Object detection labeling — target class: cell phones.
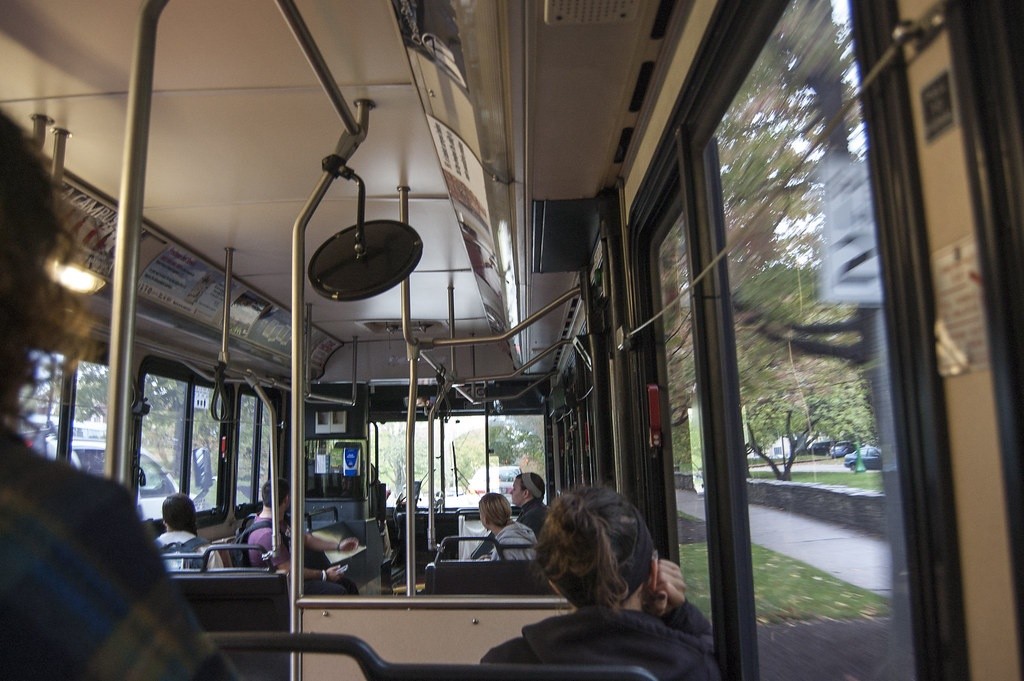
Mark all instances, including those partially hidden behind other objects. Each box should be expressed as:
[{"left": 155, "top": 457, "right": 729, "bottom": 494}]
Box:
[{"left": 336, "top": 564, "right": 348, "bottom": 572}]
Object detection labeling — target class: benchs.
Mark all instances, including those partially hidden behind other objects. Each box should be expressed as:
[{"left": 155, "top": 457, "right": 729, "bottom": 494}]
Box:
[{"left": 156, "top": 507, "right": 662, "bottom": 681}]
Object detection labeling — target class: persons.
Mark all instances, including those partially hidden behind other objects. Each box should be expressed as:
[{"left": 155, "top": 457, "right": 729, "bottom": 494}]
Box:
[
  {"left": 155, "top": 493, "right": 224, "bottom": 570},
  {"left": 479, "top": 484, "right": 719, "bottom": 681},
  {"left": 249, "top": 477, "right": 360, "bottom": 596},
  {"left": 415, "top": 396, "right": 430, "bottom": 407},
  {"left": 0, "top": 100, "right": 239, "bottom": 681},
  {"left": 476, "top": 472, "right": 549, "bottom": 560}
]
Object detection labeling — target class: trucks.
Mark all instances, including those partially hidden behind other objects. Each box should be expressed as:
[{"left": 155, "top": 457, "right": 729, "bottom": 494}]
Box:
[{"left": 25, "top": 411, "right": 214, "bottom": 520}]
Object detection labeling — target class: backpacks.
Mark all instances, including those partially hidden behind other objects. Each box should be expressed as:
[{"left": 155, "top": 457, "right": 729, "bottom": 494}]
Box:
[
  {"left": 232, "top": 521, "right": 289, "bottom": 569},
  {"left": 154, "top": 537, "right": 207, "bottom": 569}
]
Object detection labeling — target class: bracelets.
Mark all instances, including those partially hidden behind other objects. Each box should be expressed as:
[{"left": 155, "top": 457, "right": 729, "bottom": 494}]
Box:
[
  {"left": 322, "top": 569, "right": 327, "bottom": 582},
  {"left": 337, "top": 543, "right": 340, "bottom": 552}
]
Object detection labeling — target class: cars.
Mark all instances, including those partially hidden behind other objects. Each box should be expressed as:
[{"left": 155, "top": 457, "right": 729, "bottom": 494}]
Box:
[
  {"left": 807, "top": 440, "right": 834, "bottom": 456},
  {"left": 829, "top": 440, "right": 856, "bottom": 458},
  {"left": 447, "top": 486, "right": 464, "bottom": 497},
  {"left": 467, "top": 465, "right": 524, "bottom": 507},
  {"left": 843, "top": 446, "right": 883, "bottom": 471}
]
